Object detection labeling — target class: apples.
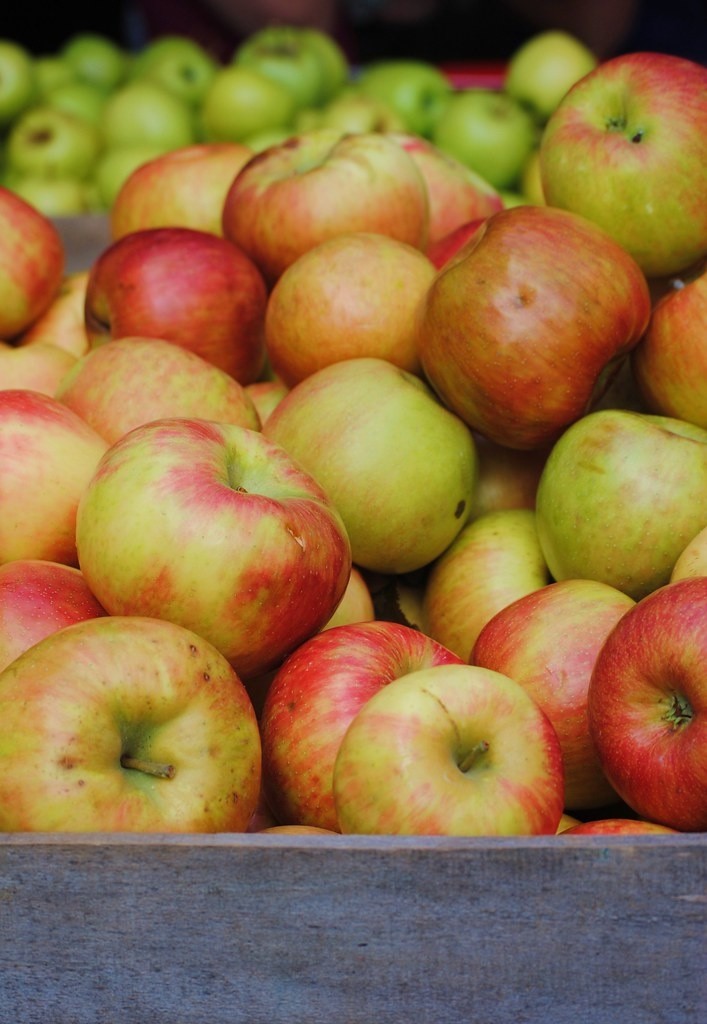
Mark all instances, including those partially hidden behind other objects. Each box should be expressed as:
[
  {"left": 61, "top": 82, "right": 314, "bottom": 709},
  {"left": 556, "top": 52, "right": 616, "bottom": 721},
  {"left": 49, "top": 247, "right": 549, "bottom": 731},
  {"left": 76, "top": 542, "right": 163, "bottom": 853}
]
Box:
[{"left": 0, "top": 23, "right": 707, "bottom": 838}]
